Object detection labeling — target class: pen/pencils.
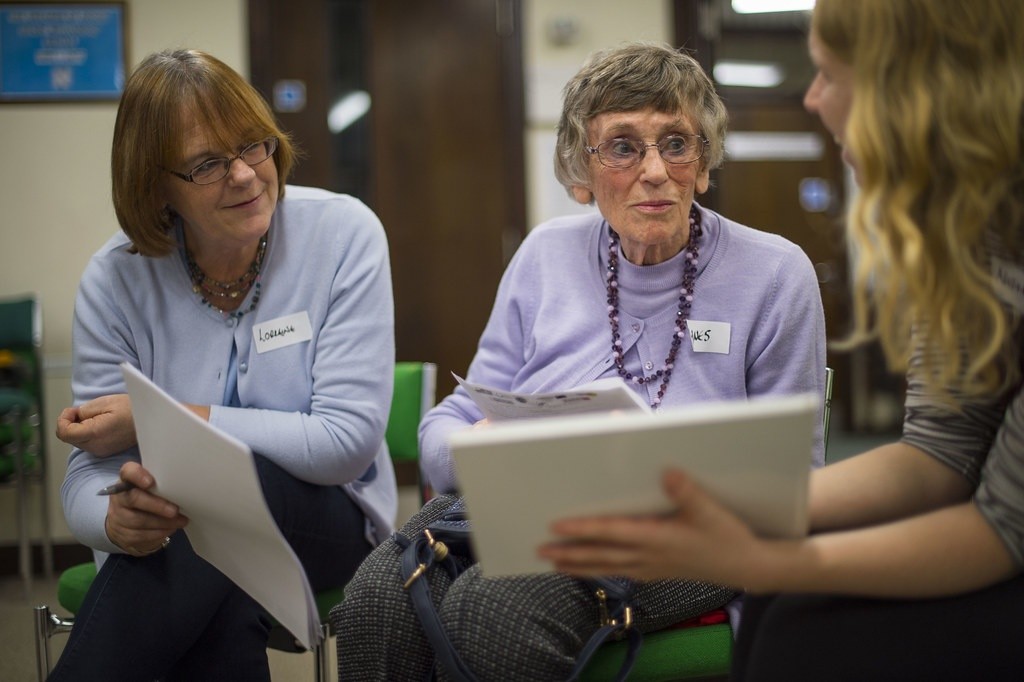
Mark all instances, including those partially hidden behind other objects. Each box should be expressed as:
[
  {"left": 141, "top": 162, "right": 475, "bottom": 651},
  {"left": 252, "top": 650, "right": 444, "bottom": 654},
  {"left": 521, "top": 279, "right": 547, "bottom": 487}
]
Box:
[{"left": 96, "top": 480, "right": 132, "bottom": 495}]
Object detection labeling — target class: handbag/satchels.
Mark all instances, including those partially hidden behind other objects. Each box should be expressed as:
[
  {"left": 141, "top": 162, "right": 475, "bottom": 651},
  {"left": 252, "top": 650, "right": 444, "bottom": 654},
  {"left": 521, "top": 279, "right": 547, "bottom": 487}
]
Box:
[{"left": 400, "top": 499, "right": 471, "bottom": 581}]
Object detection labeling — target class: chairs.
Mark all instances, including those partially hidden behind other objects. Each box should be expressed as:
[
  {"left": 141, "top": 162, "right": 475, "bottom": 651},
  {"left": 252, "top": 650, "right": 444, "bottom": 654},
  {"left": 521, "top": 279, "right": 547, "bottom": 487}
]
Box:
[
  {"left": 0, "top": 291, "right": 52, "bottom": 600},
  {"left": 33, "top": 358, "right": 836, "bottom": 681}
]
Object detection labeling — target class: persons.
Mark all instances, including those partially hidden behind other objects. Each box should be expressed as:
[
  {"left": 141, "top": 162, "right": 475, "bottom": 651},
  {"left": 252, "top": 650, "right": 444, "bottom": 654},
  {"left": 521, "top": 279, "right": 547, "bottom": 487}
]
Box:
[
  {"left": 44, "top": 49, "right": 397, "bottom": 682},
  {"left": 540, "top": 0, "right": 1024, "bottom": 682},
  {"left": 338, "top": 43, "right": 829, "bottom": 682}
]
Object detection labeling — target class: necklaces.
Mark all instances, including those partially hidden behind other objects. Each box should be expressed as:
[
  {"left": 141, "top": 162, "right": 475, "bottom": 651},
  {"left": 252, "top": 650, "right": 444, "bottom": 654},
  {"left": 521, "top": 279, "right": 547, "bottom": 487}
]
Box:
[
  {"left": 607, "top": 207, "right": 700, "bottom": 414},
  {"left": 177, "top": 221, "right": 267, "bottom": 318}
]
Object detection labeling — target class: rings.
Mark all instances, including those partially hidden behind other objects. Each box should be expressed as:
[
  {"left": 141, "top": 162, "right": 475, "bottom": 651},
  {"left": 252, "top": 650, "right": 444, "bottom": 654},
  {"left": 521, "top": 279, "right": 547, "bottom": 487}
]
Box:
[{"left": 162, "top": 537, "right": 170, "bottom": 546}]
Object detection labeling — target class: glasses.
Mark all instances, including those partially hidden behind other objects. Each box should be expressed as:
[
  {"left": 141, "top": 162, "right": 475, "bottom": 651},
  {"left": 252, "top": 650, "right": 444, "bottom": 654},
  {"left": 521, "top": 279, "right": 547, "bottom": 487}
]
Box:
[
  {"left": 585, "top": 132, "right": 710, "bottom": 169},
  {"left": 162, "top": 134, "right": 278, "bottom": 186}
]
